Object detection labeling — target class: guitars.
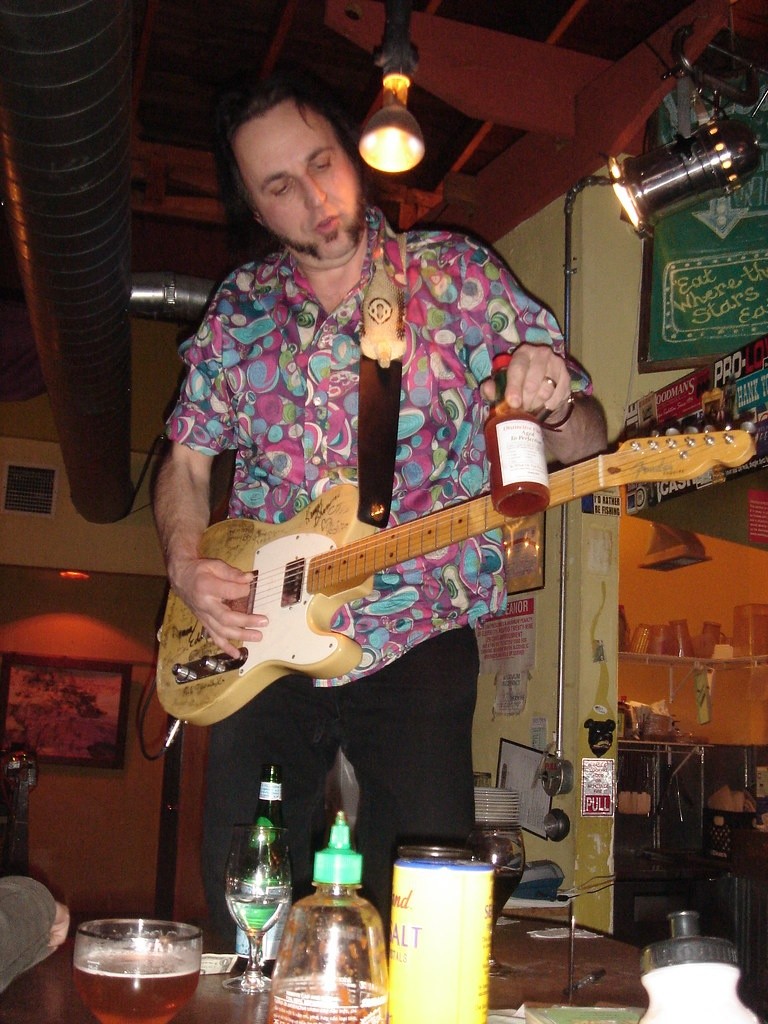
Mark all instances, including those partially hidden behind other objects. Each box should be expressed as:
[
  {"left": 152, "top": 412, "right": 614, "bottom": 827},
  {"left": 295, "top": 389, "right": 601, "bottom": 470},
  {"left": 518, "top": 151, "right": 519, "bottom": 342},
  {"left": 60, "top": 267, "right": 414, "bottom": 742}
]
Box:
[{"left": 153, "top": 420, "right": 756, "bottom": 726}]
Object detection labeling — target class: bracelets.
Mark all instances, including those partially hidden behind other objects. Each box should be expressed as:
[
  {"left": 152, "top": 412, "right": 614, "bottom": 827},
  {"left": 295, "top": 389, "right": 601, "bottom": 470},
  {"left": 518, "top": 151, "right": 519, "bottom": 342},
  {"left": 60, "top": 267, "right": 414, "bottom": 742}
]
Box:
[{"left": 542, "top": 391, "right": 575, "bottom": 429}]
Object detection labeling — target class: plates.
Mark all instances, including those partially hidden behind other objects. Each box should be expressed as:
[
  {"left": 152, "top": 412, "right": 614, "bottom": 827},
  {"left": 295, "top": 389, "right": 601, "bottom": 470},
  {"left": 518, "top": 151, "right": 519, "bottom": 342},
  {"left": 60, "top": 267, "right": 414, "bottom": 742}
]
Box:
[{"left": 474, "top": 785, "right": 521, "bottom": 821}]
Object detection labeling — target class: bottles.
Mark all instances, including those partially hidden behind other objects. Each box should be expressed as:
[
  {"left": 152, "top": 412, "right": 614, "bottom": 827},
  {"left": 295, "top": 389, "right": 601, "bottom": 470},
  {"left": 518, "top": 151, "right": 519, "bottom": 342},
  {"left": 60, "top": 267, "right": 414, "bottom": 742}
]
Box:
[
  {"left": 619, "top": 605, "right": 627, "bottom": 652},
  {"left": 698, "top": 621, "right": 723, "bottom": 658},
  {"left": 265, "top": 810, "right": 389, "bottom": 1024},
  {"left": 636, "top": 910, "right": 760, "bottom": 1024},
  {"left": 484, "top": 353, "right": 551, "bottom": 516},
  {"left": 235, "top": 764, "right": 297, "bottom": 978}
]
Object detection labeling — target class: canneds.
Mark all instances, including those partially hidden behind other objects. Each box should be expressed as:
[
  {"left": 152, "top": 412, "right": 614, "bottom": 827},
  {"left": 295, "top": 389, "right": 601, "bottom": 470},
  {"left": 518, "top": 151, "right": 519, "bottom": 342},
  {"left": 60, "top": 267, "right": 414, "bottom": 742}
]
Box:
[{"left": 386, "top": 858, "right": 494, "bottom": 1024}]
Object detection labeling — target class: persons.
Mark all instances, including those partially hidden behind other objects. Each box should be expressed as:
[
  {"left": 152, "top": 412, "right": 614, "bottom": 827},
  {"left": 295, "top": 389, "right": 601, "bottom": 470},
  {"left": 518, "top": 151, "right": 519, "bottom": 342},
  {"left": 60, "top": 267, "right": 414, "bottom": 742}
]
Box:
[{"left": 153, "top": 71, "right": 608, "bottom": 980}]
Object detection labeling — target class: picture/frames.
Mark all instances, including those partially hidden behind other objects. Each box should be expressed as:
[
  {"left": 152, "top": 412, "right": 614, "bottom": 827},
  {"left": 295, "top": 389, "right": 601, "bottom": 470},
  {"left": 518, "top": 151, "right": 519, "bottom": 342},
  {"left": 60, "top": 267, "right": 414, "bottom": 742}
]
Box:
[{"left": 0, "top": 650, "right": 133, "bottom": 770}]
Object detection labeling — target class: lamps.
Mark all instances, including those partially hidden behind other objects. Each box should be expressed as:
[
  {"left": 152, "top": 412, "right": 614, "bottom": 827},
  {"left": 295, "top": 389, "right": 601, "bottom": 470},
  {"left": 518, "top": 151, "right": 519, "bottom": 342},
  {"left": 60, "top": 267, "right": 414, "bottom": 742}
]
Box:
[
  {"left": 603, "top": 117, "right": 762, "bottom": 238},
  {"left": 354, "top": 0, "right": 424, "bottom": 173}
]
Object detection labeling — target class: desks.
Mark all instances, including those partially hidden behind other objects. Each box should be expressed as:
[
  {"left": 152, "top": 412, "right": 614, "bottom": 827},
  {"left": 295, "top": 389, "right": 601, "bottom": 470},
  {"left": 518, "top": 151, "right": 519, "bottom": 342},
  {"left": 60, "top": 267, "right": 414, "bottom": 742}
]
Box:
[{"left": 0, "top": 913, "right": 650, "bottom": 1024}]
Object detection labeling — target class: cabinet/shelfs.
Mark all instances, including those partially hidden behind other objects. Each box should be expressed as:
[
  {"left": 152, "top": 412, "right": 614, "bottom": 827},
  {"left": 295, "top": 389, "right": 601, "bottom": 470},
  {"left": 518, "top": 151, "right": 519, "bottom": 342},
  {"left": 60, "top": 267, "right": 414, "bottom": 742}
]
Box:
[{"left": 614, "top": 739, "right": 768, "bottom": 1023}]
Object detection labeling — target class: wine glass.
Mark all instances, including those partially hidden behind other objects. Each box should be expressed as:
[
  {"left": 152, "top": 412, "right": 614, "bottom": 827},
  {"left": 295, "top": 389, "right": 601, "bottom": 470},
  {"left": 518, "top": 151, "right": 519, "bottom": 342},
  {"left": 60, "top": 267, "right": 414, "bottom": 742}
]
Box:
[
  {"left": 467, "top": 826, "right": 525, "bottom": 976},
  {"left": 223, "top": 823, "right": 292, "bottom": 996}
]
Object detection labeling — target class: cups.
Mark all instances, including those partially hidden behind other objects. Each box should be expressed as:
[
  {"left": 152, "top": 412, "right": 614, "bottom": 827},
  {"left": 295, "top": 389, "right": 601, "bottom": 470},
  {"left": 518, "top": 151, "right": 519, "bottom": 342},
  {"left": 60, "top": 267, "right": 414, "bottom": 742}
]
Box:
[
  {"left": 733, "top": 604, "right": 768, "bottom": 657},
  {"left": 663, "top": 618, "right": 697, "bottom": 657},
  {"left": 384, "top": 844, "right": 496, "bottom": 1024},
  {"left": 72, "top": 917, "right": 204, "bottom": 1024},
  {"left": 629, "top": 624, "right": 652, "bottom": 655},
  {"left": 648, "top": 624, "right": 668, "bottom": 655}
]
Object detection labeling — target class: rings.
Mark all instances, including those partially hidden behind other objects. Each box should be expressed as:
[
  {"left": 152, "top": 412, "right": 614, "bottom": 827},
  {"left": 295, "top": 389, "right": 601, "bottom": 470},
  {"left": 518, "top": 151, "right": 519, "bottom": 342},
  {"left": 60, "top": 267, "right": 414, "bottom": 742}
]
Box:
[{"left": 543, "top": 376, "right": 557, "bottom": 389}]
